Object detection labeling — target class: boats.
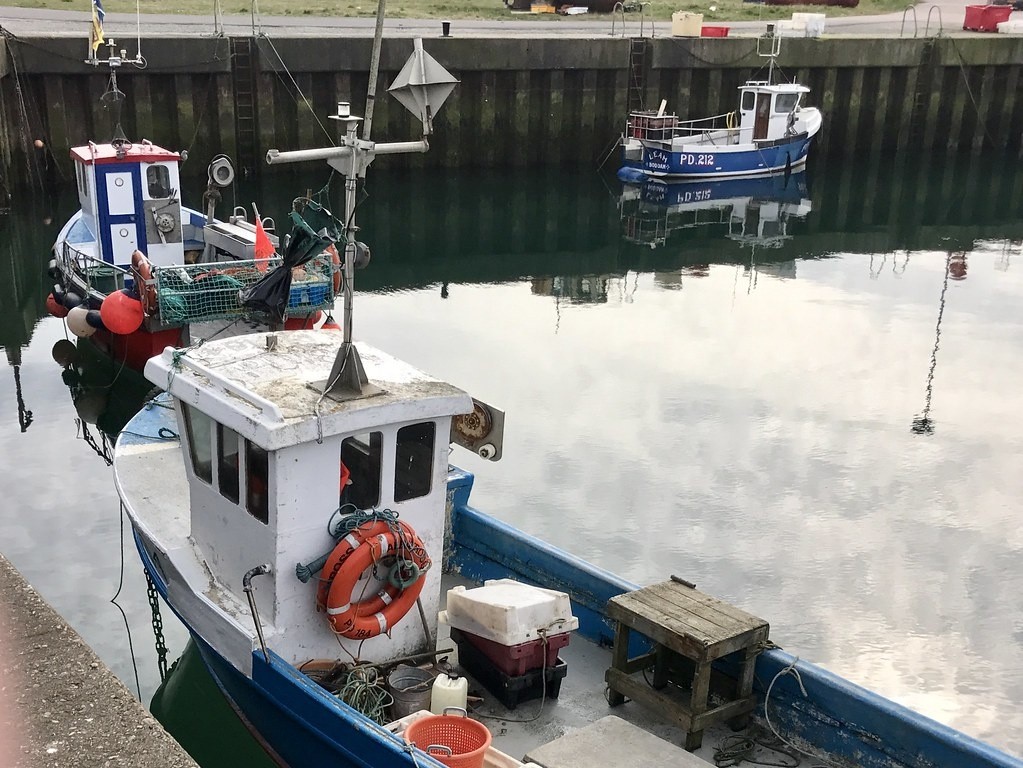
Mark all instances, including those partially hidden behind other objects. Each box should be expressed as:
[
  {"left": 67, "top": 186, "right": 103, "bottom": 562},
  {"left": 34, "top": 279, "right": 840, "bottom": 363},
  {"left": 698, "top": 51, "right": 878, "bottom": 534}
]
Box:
[
  {"left": 615, "top": 20, "right": 820, "bottom": 189},
  {"left": 45, "top": 108, "right": 347, "bottom": 385},
  {"left": 617, "top": 193, "right": 812, "bottom": 268},
  {"left": 113, "top": 98, "right": 1023, "bottom": 767}
]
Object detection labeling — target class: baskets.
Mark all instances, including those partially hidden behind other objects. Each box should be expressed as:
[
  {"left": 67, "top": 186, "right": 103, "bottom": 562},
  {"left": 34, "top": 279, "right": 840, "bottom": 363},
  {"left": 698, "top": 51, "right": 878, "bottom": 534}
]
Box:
[{"left": 404, "top": 707, "right": 492, "bottom": 768}]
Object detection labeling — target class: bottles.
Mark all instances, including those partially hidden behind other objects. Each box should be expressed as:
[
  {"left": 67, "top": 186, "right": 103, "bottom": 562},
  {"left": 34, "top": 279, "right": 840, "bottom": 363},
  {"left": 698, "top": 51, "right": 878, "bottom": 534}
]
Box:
[{"left": 430, "top": 672, "right": 469, "bottom": 716}]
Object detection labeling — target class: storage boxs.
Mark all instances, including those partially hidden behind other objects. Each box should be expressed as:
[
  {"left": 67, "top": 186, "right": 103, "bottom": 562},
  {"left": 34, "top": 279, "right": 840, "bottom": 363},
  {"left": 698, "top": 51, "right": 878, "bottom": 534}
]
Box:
[
  {"left": 701, "top": 26, "right": 731, "bottom": 38},
  {"left": 384, "top": 708, "right": 527, "bottom": 768},
  {"left": 996, "top": 21, "right": 1023, "bottom": 34},
  {"left": 672, "top": 10, "right": 704, "bottom": 36},
  {"left": 776, "top": 12, "right": 827, "bottom": 39},
  {"left": 439, "top": 578, "right": 580, "bottom": 709},
  {"left": 962, "top": 4, "right": 1014, "bottom": 33},
  {"left": 291, "top": 286, "right": 328, "bottom": 308}
]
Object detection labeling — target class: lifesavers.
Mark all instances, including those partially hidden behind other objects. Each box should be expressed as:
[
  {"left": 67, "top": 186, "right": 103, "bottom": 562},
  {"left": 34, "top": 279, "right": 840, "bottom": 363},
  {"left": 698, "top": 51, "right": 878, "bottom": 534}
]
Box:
[
  {"left": 325, "top": 243, "right": 341, "bottom": 298},
  {"left": 317, "top": 519, "right": 416, "bottom": 617},
  {"left": 132, "top": 249, "right": 156, "bottom": 316},
  {"left": 327, "top": 531, "right": 431, "bottom": 639}
]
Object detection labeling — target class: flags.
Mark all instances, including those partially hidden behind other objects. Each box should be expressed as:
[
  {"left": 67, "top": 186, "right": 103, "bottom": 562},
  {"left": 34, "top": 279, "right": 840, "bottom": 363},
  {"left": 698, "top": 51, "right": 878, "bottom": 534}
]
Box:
[{"left": 254, "top": 214, "right": 275, "bottom": 271}]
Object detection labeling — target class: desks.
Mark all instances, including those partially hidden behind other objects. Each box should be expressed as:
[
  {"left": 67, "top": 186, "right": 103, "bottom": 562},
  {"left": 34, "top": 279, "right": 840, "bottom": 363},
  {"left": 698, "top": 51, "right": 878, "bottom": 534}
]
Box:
[{"left": 605, "top": 578, "right": 769, "bottom": 753}]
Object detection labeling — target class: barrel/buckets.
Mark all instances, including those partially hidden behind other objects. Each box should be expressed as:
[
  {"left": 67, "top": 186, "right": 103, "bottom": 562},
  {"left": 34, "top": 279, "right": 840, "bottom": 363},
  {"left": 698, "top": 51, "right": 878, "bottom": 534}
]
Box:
[{"left": 386, "top": 664, "right": 436, "bottom": 721}]
